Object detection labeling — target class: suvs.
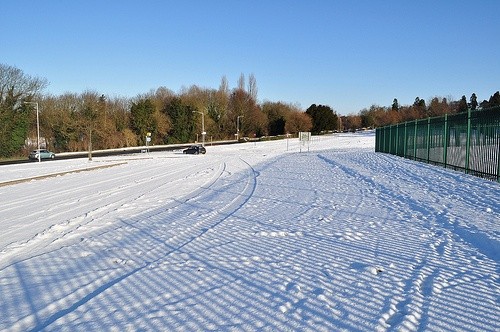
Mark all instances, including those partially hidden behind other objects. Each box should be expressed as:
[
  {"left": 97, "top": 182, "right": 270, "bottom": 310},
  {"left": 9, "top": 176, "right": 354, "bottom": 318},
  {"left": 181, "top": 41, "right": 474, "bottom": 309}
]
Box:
[{"left": 183, "top": 145, "right": 206, "bottom": 155}]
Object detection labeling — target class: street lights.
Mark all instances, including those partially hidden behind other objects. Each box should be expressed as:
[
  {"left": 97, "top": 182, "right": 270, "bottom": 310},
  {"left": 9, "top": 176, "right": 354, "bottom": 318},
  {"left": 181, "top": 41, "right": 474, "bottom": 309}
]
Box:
[
  {"left": 237, "top": 116, "right": 244, "bottom": 141},
  {"left": 23, "top": 101, "right": 41, "bottom": 163},
  {"left": 192, "top": 111, "right": 205, "bottom": 147}
]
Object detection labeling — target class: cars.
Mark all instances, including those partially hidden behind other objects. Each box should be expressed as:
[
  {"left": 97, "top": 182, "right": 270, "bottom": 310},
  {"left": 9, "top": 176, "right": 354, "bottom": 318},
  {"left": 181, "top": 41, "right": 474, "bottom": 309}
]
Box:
[{"left": 29, "top": 149, "right": 55, "bottom": 160}]
